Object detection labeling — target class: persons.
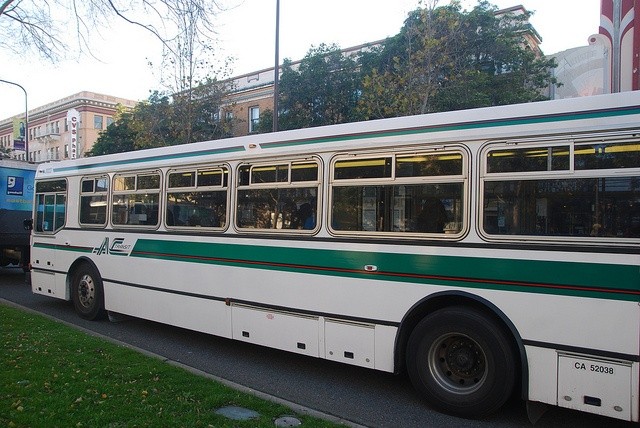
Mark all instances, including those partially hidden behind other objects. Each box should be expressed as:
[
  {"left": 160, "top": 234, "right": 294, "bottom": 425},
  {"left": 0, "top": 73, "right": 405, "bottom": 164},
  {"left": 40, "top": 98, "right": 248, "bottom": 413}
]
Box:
[{"left": 304, "top": 196, "right": 339, "bottom": 229}]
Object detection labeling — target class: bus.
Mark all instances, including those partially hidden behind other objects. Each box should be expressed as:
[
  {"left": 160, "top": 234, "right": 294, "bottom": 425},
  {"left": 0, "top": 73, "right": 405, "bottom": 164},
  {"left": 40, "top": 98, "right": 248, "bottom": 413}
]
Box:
[{"left": 28, "top": 90, "right": 640, "bottom": 423}]
